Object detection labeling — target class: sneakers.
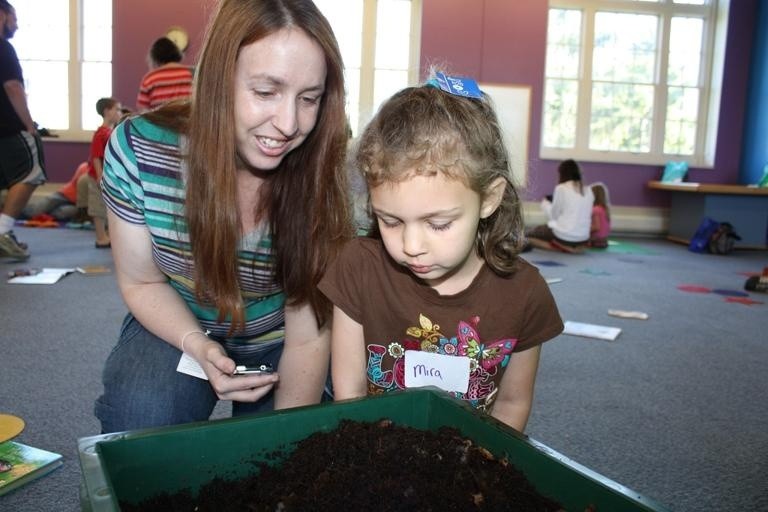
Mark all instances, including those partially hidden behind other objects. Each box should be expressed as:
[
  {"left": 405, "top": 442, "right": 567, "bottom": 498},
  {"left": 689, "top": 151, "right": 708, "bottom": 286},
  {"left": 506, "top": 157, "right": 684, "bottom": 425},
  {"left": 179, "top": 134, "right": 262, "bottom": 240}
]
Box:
[
  {"left": 96, "top": 239, "right": 111, "bottom": 249},
  {"left": 0, "top": 230, "right": 31, "bottom": 261}
]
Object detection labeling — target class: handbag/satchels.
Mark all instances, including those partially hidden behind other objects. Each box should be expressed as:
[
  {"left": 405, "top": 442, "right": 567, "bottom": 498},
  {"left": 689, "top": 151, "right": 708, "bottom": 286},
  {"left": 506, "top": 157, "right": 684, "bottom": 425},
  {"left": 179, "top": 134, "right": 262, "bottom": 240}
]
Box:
[{"left": 688, "top": 216, "right": 740, "bottom": 255}]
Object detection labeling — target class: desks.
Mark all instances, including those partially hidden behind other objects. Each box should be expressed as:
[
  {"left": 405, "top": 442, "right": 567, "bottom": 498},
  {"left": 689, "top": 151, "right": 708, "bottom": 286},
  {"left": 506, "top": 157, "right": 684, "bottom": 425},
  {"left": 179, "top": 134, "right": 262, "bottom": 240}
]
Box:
[{"left": 646, "top": 180, "right": 767, "bottom": 250}]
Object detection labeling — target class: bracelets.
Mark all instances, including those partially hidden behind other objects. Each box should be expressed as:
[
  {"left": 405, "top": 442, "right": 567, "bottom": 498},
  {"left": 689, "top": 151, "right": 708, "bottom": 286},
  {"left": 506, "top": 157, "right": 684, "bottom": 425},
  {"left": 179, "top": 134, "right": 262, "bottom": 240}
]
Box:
[{"left": 179, "top": 326, "right": 209, "bottom": 356}]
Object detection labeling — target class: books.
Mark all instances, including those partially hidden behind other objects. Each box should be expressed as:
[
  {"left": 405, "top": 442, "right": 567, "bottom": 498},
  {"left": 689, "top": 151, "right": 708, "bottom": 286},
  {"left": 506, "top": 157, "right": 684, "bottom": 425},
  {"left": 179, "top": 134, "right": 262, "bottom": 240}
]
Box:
[
  {"left": 6, "top": 267, "right": 76, "bottom": 285},
  {"left": 0, "top": 438, "right": 64, "bottom": 498}
]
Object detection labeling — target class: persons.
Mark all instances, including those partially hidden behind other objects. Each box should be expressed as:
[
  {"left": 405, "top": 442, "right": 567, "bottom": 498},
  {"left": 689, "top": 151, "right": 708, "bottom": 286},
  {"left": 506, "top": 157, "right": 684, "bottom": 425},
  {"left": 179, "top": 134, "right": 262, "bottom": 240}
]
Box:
[
  {"left": 133, "top": 35, "right": 198, "bottom": 112},
  {"left": 84, "top": 97, "right": 126, "bottom": 248},
  {"left": 1, "top": 157, "right": 96, "bottom": 227},
  {"left": 587, "top": 181, "right": 611, "bottom": 251},
  {"left": 91, "top": 0, "right": 354, "bottom": 431},
  {"left": 521, "top": 158, "right": 594, "bottom": 254},
  {"left": 1, "top": 0, "right": 45, "bottom": 265},
  {"left": 309, "top": 64, "right": 565, "bottom": 442}
]
